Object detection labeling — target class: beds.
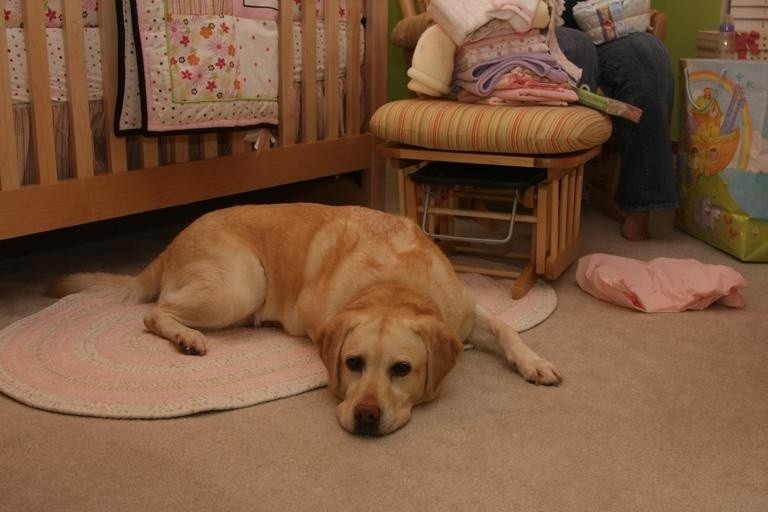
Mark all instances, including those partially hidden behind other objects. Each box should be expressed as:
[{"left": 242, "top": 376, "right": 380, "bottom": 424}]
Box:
[{"left": 0, "top": 2, "right": 393, "bottom": 258}]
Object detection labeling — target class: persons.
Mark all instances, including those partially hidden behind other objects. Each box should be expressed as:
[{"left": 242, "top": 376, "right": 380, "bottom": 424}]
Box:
[{"left": 546, "top": 0, "right": 683, "bottom": 242}]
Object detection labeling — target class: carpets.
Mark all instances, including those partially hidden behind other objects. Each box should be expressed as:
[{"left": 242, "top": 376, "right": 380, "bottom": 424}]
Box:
[{"left": 2, "top": 263, "right": 559, "bottom": 419}]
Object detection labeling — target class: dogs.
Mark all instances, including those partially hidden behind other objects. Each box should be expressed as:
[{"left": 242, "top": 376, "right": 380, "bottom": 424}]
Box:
[{"left": 41, "top": 197, "right": 565, "bottom": 440}]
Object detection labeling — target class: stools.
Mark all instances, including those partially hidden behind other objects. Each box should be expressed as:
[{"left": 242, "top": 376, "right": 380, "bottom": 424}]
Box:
[{"left": 372, "top": 94, "right": 617, "bottom": 287}]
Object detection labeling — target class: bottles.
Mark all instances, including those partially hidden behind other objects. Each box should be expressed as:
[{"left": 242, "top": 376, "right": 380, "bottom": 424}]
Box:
[{"left": 719, "top": 23, "right": 736, "bottom": 60}]
